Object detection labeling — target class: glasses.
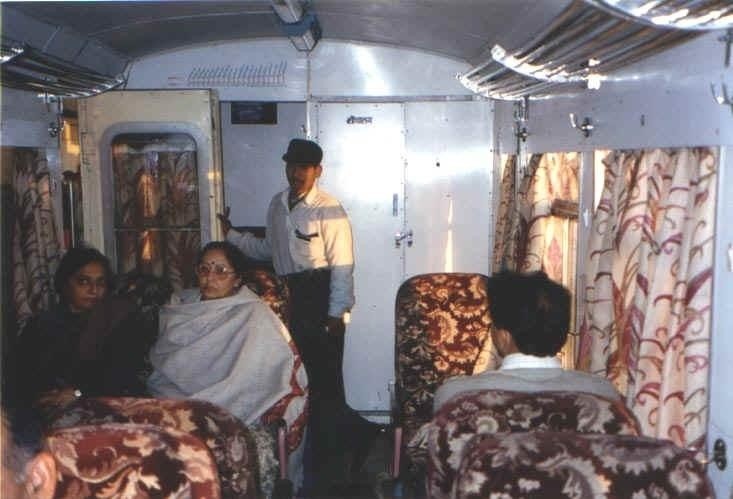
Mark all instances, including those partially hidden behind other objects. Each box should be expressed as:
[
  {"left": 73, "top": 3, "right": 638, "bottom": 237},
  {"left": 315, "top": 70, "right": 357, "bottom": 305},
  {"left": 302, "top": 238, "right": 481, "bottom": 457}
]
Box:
[{"left": 196, "top": 264, "right": 238, "bottom": 276}]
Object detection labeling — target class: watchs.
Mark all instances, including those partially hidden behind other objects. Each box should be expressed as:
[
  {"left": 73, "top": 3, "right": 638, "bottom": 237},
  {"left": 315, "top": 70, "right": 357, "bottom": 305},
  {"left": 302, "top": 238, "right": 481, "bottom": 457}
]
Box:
[{"left": 73, "top": 386, "right": 81, "bottom": 397}]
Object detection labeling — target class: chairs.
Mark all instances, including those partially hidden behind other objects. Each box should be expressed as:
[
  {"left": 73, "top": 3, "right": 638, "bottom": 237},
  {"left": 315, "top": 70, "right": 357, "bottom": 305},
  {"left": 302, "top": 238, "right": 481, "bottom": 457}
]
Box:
[
  {"left": 396, "top": 274, "right": 715, "bottom": 498},
  {"left": 2, "top": 267, "right": 308, "bottom": 495}
]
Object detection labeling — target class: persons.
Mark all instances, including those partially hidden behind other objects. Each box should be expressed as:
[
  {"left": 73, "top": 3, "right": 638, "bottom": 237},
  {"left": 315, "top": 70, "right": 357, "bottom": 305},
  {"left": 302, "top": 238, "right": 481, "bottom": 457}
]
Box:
[
  {"left": 148, "top": 241, "right": 296, "bottom": 497},
  {"left": 6, "top": 248, "right": 150, "bottom": 440},
  {"left": 424, "top": 271, "right": 627, "bottom": 428},
  {"left": 216, "top": 140, "right": 357, "bottom": 453}
]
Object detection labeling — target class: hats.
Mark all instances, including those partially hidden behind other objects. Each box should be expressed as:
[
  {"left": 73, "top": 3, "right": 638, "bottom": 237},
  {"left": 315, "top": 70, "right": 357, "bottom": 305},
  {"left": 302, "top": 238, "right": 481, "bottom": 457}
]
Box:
[{"left": 282, "top": 139, "right": 322, "bottom": 164}]
